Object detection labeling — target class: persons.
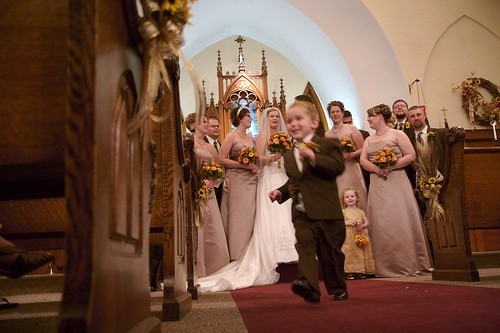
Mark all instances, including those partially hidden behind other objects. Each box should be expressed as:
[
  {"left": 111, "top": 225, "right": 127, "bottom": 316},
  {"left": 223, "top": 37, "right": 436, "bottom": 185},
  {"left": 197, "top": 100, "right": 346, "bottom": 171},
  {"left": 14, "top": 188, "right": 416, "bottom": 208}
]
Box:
[
  {"left": 185, "top": 107, "right": 300, "bottom": 292},
  {"left": 0, "top": 236, "right": 54, "bottom": 311},
  {"left": 341, "top": 187, "right": 376, "bottom": 280},
  {"left": 392, "top": 99, "right": 435, "bottom": 218},
  {"left": 269, "top": 95, "right": 370, "bottom": 303},
  {"left": 359, "top": 104, "right": 435, "bottom": 278}
]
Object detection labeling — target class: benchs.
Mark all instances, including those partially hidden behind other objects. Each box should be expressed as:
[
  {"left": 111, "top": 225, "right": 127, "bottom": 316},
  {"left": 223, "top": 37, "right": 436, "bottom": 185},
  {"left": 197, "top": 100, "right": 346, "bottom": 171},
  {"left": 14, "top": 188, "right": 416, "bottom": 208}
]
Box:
[
  {"left": 430, "top": 127, "right": 500, "bottom": 282},
  {"left": 0, "top": 0, "right": 200, "bottom": 333}
]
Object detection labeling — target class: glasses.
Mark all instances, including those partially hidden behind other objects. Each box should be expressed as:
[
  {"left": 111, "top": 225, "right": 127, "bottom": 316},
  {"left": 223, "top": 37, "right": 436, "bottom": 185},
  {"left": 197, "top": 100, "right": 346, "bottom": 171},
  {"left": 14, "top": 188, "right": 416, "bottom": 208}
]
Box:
[
  {"left": 245, "top": 113, "right": 251, "bottom": 117},
  {"left": 394, "top": 104, "right": 407, "bottom": 108}
]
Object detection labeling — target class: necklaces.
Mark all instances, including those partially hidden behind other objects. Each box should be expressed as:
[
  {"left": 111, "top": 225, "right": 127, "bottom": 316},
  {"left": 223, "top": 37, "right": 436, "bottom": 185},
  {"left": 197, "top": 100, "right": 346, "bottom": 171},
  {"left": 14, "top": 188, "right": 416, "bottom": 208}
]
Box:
[
  {"left": 195, "top": 138, "right": 206, "bottom": 146},
  {"left": 376, "top": 128, "right": 389, "bottom": 136},
  {"left": 332, "top": 125, "right": 343, "bottom": 134},
  {"left": 235, "top": 130, "right": 247, "bottom": 139}
]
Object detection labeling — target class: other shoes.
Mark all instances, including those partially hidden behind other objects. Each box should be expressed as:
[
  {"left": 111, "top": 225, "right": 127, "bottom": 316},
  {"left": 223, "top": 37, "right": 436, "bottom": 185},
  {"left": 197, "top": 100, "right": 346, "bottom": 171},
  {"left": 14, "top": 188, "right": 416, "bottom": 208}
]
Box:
[
  {"left": 291, "top": 281, "right": 320, "bottom": 304},
  {"left": 333, "top": 289, "right": 348, "bottom": 300},
  {"left": 347, "top": 272, "right": 367, "bottom": 279}
]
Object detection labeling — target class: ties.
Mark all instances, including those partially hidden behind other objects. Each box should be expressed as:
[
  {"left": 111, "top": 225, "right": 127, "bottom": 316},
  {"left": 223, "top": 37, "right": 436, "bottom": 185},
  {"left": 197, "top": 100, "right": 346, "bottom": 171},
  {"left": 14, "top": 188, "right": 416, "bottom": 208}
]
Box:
[
  {"left": 214, "top": 141, "right": 219, "bottom": 153},
  {"left": 295, "top": 141, "right": 305, "bottom": 166},
  {"left": 398, "top": 123, "right": 403, "bottom": 131},
  {"left": 417, "top": 132, "right": 424, "bottom": 148}
]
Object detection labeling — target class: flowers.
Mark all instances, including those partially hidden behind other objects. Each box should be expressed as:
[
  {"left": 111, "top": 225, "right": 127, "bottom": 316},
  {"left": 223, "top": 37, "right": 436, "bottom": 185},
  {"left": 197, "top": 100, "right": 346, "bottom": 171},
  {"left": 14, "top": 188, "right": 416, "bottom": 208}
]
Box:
[
  {"left": 415, "top": 175, "right": 446, "bottom": 223},
  {"left": 341, "top": 137, "right": 356, "bottom": 156},
  {"left": 266, "top": 131, "right": 293, "bottom": 168},
  {"left": 405, "top": 122, "right": 410, "bottom": 128},
  {"left": 238, "top": 146, "right": 260, "bottom": 176},
  {"left": 355, "top": 222, "right": 369, "bottom": 247},
  {"left": 373, "top": 144, "right": 399, "bottom": 180},
  {"left": 195, "top": 160, "right": 226, "bottom": 232}
]
